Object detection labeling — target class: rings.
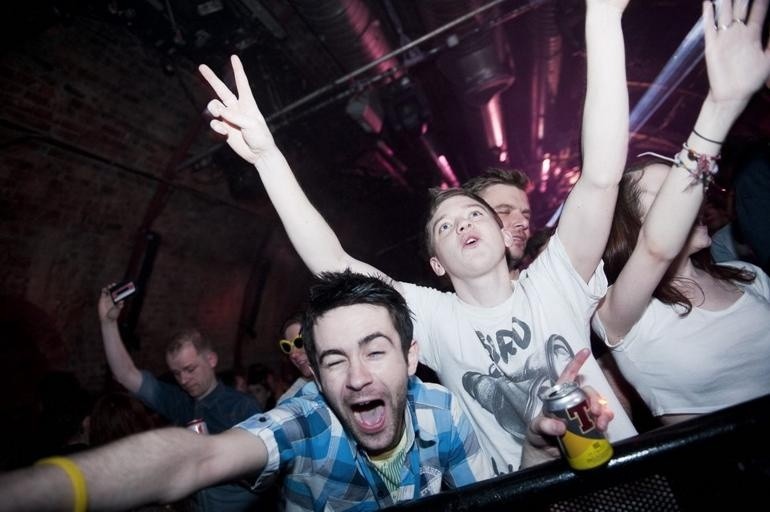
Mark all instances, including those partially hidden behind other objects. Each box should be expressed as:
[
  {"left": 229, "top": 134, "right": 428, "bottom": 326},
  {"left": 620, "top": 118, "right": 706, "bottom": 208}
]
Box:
[{"left": 714, "top": 17, "right": 749, "bottom": 30}]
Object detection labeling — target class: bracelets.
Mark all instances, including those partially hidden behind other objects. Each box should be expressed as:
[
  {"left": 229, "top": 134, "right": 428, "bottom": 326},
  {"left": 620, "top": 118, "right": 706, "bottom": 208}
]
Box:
[
  {"left": 674, "top": 127, "right": 726, "bottom": 188},
  {"left": 35, "top": 455, "right": 87, "bottom": 512}
]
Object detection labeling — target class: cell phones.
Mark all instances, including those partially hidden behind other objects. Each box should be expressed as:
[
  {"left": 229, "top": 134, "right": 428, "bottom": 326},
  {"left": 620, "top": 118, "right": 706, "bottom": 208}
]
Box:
[{"left": 109, "top": 276, "right": 137, "bottom": 304}]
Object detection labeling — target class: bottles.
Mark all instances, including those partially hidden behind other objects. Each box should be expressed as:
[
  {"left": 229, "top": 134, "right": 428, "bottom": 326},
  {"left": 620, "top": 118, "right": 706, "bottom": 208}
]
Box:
[
  {"left": 185, "top": 418, "right": 208, "bottom": 435},
  {"left": 539, "top": 382, "right": 614, "bottom": 472}
]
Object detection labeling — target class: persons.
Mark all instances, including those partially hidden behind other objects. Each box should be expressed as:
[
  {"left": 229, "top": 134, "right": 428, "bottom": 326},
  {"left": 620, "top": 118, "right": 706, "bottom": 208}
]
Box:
[
  {"left": 218, "top": 365, "right": 294, "bottom": 412},
  {"left": 197, "top": 0, "right": 637, "bottom": 472},
  {"left": 1, "top": 269, "right": 615, "bottom": 512},
  {"left": 591, "top": 0, "right": 770, "bottom": 425},
  {"left": 277, "top": 303, "right": 315, "bottom": 404},
  {"left": 459, "top": 170, "right": 532, "bottom": 274},
  {"left": 98, "top": 281, "right": 266, "bottom": 512}
]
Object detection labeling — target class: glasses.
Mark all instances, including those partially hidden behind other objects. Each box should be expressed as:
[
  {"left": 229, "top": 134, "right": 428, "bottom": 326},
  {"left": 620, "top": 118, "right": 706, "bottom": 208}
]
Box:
[{"left": 279, "top": 335, "right": 305, "bottom": 355}]
